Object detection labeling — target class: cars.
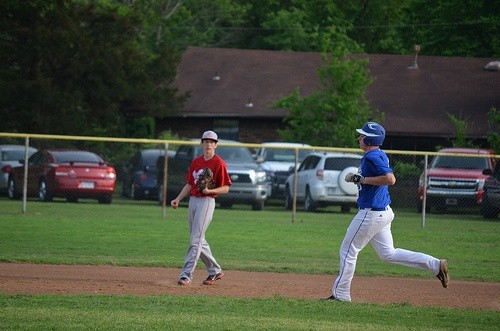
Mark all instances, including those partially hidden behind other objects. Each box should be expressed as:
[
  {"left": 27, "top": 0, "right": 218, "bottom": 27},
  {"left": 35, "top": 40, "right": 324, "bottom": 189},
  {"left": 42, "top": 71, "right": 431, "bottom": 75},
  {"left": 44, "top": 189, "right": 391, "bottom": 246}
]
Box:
[
  {"left": 0, "top": 144, "right": 40, "bottom": 191},
  {"left": 482, "top": 160, "right": 500, "bottom": 218},
  {"left": 121, "top": 150, "right": 178, "bottom": 199},
  {"left": 7, "top": 149, "right": 118, "bottom": 203}
]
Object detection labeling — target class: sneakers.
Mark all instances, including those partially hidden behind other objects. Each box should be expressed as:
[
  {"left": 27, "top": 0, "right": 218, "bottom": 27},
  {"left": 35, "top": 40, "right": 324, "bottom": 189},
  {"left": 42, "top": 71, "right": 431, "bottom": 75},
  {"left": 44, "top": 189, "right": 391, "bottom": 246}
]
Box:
[
  {"left": 203, "top": 271, "right": 224, "bottom": 284},
  {"left": 436, "top": 259, "right": 449, "bottom": 288},
  {"left": 321, "top": 295, "right": 340, "bottom": 301},
  {"left": 178, "top": 277, "right": 190, "bottom": 286}
]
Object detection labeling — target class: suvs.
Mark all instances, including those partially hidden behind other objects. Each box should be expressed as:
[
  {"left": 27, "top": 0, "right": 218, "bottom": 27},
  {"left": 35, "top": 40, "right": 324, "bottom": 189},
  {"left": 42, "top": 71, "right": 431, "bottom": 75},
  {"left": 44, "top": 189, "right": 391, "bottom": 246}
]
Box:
[
  {"left": 418, "top": 148, "right": 496, "bottom": 213},
  {"left": 252, "top": 142, "right": 316, "bottom": 196},
  {"left": 284, "top": 151, "right": 369, "bottom": 213},
  {"left": 158, "top": 137, "right": 272, "bottom": 211}
]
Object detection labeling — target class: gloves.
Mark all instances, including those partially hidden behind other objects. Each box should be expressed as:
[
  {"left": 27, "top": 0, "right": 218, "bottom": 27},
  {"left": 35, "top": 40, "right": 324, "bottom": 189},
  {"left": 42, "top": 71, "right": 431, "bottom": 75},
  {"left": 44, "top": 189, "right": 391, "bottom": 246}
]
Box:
[{"left": 345, "top": 173, "right": 363, "bottom": 185}]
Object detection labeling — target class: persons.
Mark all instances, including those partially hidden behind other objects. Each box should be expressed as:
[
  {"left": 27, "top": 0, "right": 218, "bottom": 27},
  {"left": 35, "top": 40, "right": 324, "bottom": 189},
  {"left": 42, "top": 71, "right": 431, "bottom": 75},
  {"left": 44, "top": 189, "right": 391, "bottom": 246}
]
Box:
[
  {"left": 318, "top": 122, "right": 448, "bottom": 302},
  {"left": 171, "top": 131, "right": 232, "bottom": 285}
]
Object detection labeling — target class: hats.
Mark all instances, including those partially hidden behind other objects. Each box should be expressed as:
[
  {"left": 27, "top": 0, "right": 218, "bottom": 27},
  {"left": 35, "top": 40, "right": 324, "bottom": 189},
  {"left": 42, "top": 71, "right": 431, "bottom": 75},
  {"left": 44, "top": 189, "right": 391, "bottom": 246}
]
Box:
[{"left": 201, "top": 130, "right": 218, "bottom": 142}]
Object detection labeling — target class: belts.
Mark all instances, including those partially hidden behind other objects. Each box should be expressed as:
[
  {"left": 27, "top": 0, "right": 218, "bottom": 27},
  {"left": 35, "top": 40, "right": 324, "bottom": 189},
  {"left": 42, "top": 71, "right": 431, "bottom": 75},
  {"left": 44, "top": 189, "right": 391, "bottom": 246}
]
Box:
[{"left": 360, "top": 207, "right": 385, "bottom": 211}]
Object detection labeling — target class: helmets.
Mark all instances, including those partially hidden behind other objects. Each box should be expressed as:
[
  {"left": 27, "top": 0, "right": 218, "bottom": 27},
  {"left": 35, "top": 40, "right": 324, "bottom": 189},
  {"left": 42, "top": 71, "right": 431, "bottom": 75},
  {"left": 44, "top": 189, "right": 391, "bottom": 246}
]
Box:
[{"left": 356, "top": 122, "right": 385, "bottom": 146}]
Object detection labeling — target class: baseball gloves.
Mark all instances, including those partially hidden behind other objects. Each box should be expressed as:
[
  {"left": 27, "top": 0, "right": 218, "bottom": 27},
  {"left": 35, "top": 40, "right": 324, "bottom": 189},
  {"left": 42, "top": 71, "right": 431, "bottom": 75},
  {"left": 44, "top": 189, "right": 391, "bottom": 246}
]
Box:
[{"left": 198, "top": 167, "right": 216, "bottom": 193}]
